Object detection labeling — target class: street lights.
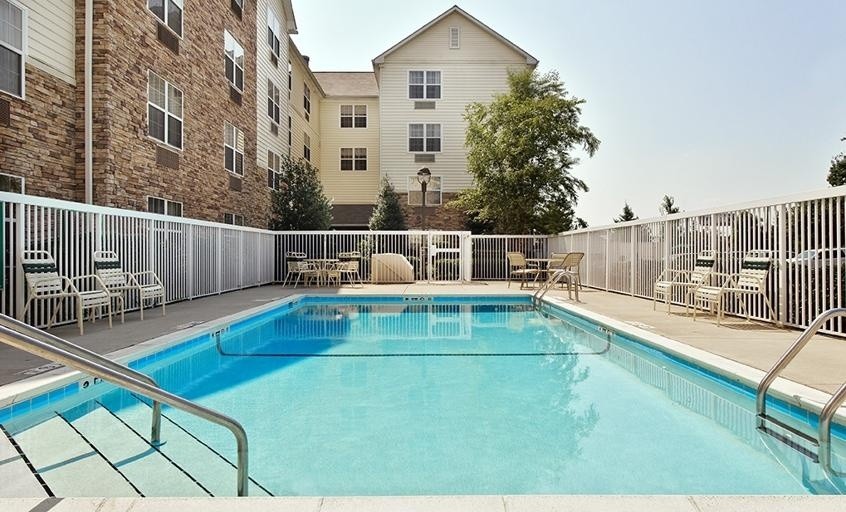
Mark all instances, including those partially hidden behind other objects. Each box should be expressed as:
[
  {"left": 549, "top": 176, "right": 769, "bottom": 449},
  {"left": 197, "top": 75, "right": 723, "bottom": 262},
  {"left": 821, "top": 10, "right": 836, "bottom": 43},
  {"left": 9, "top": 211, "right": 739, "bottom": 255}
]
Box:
[{"left": 416, "top": 168, "right": 431, "bottom": 230}]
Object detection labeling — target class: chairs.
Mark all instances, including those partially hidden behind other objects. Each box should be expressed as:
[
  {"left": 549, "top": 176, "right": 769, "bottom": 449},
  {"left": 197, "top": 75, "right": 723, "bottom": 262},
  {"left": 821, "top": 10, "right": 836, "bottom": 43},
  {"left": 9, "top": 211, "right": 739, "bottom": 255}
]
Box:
[
  {"left": 506, "top": 251, "right": 585, "bottom": 295},
  {"left": 651, "top": 248, "right": 782, "bottom": 329},
  {"left": 281, "top": 252, "right": 364, "bottom": 289},
  {"left": 18, "top": 247, "right": 169, "bottom": 337}
]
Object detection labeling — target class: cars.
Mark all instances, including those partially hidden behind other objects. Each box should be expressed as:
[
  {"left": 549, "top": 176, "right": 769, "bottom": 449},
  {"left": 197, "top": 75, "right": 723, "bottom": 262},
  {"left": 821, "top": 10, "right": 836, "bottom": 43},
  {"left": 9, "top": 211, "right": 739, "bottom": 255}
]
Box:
[{"left": 672, "top": 248, "right": 845, "bottom": 269}]
